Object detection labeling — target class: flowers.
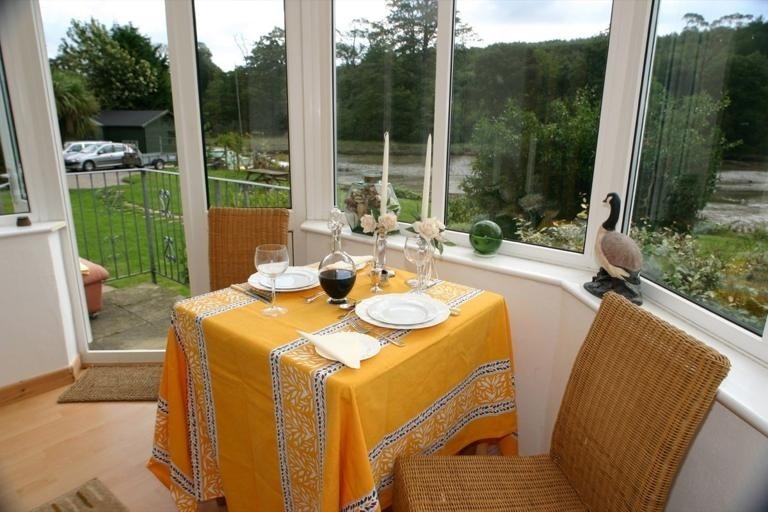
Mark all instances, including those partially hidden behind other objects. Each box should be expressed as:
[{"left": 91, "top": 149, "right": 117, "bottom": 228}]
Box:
[
  {"left": 359, "top": 212, "right": 400, "bottom": 236},
  {"left": 405, "top": 217, "right": 457, "bottom": 256}
]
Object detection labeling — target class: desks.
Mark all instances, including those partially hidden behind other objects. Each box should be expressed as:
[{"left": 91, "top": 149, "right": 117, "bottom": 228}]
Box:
[{"left": 243, "top": 168, "right": 288, "bottom": 187}]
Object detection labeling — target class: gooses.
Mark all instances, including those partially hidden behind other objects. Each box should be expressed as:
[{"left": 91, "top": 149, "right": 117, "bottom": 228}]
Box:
[{"left": 594, "top": 191, "right": 649, "bottom": 286}]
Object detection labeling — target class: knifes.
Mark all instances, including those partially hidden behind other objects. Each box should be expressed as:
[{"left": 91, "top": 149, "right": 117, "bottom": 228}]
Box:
[{"left": 230, "top": 283, "right": 272, "bottom": 304}]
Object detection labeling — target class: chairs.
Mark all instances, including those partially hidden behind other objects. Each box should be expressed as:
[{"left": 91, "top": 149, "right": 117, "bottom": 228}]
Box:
[
  {"left": 390, "top": 291, "right": 732, "bottom": 512},
  {"left": 208, "top": 207, "right": 290, "bottom": 294}
]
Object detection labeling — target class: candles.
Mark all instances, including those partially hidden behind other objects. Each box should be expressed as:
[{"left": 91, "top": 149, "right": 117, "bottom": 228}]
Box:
[
  {"left": 380, "top": 131, "right": 389, "bottom": 216},
  {"left": 420, "top": 134, "right": 432, "bottom": 220}
]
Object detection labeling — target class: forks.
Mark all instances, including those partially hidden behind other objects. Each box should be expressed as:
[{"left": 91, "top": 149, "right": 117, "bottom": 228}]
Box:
[{"left": 347, "top": 317, "right": 406, "bottom": 347}]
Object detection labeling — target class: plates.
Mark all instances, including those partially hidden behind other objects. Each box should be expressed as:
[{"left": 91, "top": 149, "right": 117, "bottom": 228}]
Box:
[
  {"left": 315, "top": 332, "right": 381, "bottom": 361},
  {"left": 354, "top": 294, "right": 450, "bottom": 330},
  {"left": 248, "top": 266, "right": 321, "bottom": 293}
]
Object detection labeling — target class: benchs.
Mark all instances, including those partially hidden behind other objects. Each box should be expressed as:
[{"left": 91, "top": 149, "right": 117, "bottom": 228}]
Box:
[{"left": 265, "top": 176, "right": 287, "bottom": 186}]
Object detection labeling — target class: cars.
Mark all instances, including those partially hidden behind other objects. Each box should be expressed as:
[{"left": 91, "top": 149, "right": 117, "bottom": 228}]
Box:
[{"left": 62, "top": 140, "right": 288, "bottom": 173}]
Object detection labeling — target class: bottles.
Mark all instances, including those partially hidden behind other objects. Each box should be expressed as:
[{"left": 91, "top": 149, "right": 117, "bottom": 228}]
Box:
[
  {"left": 344, "top": 172, "right": 402, "bottom": 237},
  {"left": 318, "top": 206, "right": 357, "bottom": 306}
]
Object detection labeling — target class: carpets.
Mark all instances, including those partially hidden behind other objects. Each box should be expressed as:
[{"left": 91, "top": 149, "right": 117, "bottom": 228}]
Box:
[
  {"left": 27, "top": 478, "right": 130, "bottom": 512},
  {"left": 57, "top": 364, "right": 163, "bottom": 403}
]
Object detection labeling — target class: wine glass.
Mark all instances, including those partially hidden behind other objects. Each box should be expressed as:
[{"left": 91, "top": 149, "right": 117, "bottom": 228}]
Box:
[
  {"left": 404, "top": 236, "right": 436, "bottom": 298},
  {"left": 254, "top": 244, "right": 289, "bottom": 318}
]
